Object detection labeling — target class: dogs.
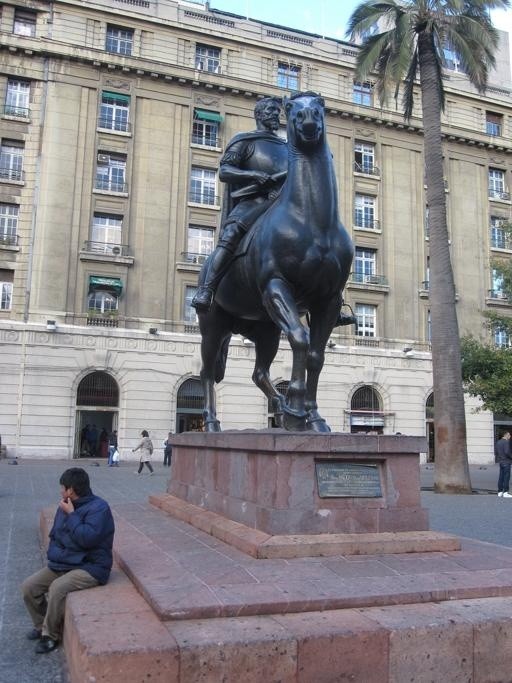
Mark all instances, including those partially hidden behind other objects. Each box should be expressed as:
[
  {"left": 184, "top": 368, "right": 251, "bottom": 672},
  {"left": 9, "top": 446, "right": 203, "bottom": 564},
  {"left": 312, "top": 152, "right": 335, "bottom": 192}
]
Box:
[{"left": 192, "top": 89, "right": 358, "bottom": 432}]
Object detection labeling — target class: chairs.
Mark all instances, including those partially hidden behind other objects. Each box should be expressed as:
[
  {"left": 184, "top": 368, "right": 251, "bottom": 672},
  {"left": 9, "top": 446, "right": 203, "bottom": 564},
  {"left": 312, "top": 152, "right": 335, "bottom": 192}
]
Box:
[{"left": 498, "top": 491, "right": 512, "bottom": 498}]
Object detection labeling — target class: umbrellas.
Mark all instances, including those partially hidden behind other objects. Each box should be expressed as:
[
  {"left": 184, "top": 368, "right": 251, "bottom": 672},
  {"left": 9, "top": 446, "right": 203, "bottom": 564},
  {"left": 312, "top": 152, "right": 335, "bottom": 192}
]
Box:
[{"left": 26, "top": 626, "right": 59, "bottom": 654}]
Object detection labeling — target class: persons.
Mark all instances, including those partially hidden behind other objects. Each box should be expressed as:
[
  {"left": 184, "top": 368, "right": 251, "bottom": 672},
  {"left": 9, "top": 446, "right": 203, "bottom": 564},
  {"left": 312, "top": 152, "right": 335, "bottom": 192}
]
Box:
[
  {"left": 23, "top": 469, "right": 115, "bottom": 653},
  {"left": 81, "top": 424, "right": 119, "bottom": 466},
  {"left": 190, "top": 96, "right": 360, "bottom": 327},
  {"left": 132, "top": 430, "right": 154, "bottom": 476},
  {"left": 495, "top": 431, "right": 512, "bottom": 498},
  {"left": 163, "top": 432, "right": 172, "bottom": 466}
]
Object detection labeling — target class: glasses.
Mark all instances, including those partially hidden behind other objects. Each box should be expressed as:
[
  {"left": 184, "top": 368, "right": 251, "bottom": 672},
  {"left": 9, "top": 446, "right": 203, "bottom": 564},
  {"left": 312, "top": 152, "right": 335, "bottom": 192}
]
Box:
[
  {"left": 326, "top": 339, "right": 336, "bottom": 348},
  {"left": 401, "top": 346, "right": 415, "bottom": 358},
  {"left": 147, "top": 327, "right": 160, "bottom": 336},
  {"left": 45, "top": 320, "right": 56, "bottom": 330}
]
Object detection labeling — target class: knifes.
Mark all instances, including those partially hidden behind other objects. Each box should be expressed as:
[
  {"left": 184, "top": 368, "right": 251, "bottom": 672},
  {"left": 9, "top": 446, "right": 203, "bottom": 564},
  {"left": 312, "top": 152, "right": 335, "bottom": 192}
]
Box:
[
  {"left": 193, "top": 254, "right": 205, "bottom": 263},
  {"left": 500, "top": 191, "right": 509, "bottom": 199},
  {"left": 365, "top": 275, "right": 380, "bottom": 283},
  {"left": 495, "top": 219, "right": 510, "bottom": 228},
  {"left": 96, "top": 153, "right": 109, "bottom": 164},
  {"left": 497, "top": 291, "right": 508, "bottom": 298},
  {"left": 109, "top": 245, "right": 121, "bottom": 255}
]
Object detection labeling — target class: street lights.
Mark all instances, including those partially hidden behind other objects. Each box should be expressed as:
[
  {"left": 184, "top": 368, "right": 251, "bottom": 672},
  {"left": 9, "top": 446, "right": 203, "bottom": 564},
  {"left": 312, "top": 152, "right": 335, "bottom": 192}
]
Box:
[{"left": 113, "top": 450, "right": 120, "bottom": 462}]
